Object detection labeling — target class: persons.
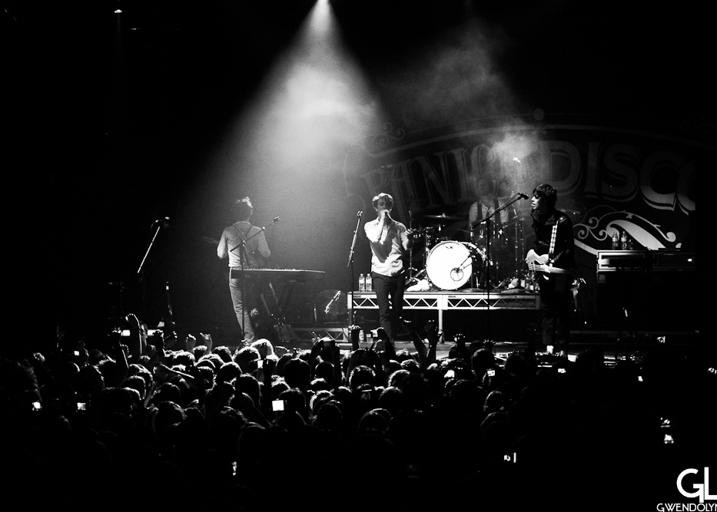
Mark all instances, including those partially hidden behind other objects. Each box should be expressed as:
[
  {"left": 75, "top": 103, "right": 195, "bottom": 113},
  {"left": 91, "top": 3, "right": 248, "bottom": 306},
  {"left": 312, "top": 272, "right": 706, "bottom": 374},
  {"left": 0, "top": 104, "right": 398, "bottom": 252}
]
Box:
[
  {"left": 216, "top": 195, "right": 271, "bottom": 348},
  {"left": 525, "top": 185, "right": 577, "bottom": 348},
  {"left": 362, "top": 191, "right": 409, "bottom": 355},
  {"left": 467, "top": 174, "right": 514, "bottom": 235}
]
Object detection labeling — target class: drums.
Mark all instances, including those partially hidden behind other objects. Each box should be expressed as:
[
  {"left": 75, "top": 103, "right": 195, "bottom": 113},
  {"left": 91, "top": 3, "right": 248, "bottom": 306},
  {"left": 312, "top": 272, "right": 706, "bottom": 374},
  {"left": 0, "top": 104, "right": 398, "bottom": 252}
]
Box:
[
  {"left": 504, "top": 216, "right": 529, "bottom": 240},
  {"left": 425, "top": 240, "right": 486, "bottom": 291},
  {"left": 472, "top": 219, "right": 501, "bottom": 246}
]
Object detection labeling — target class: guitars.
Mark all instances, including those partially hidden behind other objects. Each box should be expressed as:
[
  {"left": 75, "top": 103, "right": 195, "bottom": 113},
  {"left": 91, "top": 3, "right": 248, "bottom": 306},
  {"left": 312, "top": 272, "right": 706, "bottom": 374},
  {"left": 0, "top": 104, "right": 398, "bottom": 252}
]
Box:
[{"left": 525, "top": 248, "right": 589, "bottom": 288}]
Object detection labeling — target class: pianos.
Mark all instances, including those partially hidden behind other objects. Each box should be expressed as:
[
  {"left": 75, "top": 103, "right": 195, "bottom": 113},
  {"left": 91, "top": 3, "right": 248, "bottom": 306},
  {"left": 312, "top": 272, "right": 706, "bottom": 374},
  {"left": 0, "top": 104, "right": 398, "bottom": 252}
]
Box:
[{"left": 230, "top": 266, "right": 329, "bottom": 284}]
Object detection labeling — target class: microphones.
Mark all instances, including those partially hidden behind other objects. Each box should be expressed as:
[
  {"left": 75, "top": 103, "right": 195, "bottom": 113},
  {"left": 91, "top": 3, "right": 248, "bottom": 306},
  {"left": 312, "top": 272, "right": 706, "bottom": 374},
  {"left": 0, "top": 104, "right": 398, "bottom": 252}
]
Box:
[
  {"left": 516, "top": 192, "right": 529, "bottom": 201},
  {"left": 153, "top": 215, "right": 171, "bottom": 226},
  {"left": 454, "top": 267, "right": 460, "bottom": 274},
  {"left": 385, "top": 212, "right": 389, "bottom": 223},
  {"left": 356, "top": 209, "right": 365, "bottom": 218},
  {"left": 265, "top": 214, "right": 281, "bottom": 228}
]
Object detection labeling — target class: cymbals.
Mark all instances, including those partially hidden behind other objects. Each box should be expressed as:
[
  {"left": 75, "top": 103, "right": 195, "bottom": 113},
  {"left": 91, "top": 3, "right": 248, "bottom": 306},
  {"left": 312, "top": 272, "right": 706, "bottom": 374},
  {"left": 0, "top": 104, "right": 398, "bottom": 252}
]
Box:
[{"left": 426, "top": 214, "right": 463, "bottom": 220}]
